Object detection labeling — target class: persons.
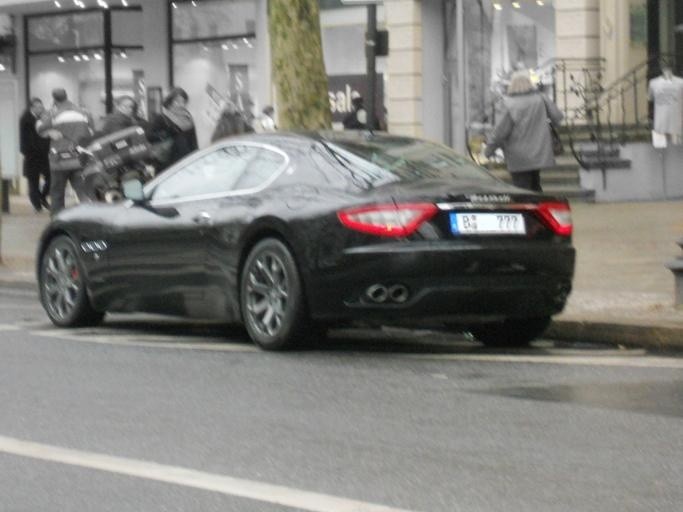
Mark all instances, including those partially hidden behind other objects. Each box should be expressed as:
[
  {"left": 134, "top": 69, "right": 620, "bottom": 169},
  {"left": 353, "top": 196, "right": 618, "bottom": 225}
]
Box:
[
  {"left": 343, "top": 97, "right": 380, "bottom": 129},
  {"left": 483, "top": 71, "right": 564, "bottom": 191},
  {"left": 20, "top": 89, "right": 275, "bottom": 217},
  {"left": 647, "top": 70, "right": 682, "bottom": 136}
]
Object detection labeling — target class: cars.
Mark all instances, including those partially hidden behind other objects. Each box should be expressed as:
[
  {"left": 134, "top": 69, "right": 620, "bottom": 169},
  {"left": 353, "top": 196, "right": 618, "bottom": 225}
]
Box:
[{"left": 35, "top": 130, "right": 575, "bottom": 351}]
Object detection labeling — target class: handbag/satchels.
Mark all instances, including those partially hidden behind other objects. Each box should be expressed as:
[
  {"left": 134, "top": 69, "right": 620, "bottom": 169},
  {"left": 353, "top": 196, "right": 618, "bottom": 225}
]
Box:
[{"left": 537, "top": 93, "right": 564, "bottom": 156}]
